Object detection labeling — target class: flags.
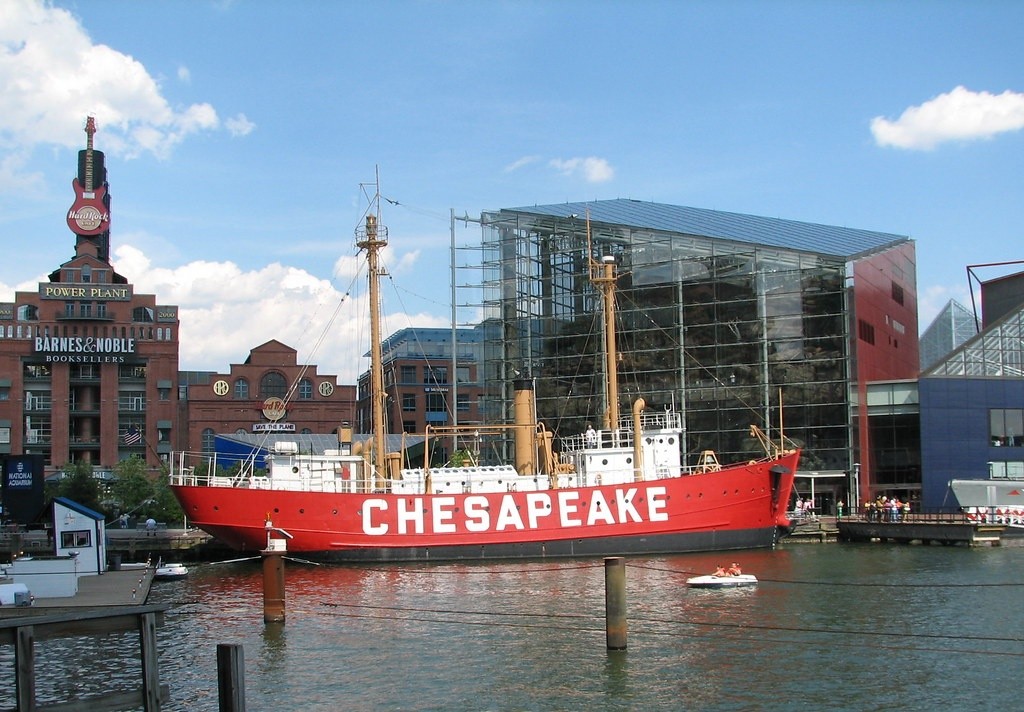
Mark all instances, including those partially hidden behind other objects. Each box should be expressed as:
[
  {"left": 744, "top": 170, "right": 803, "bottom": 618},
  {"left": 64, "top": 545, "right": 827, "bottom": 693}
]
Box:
[{"left": 124, "top": 423, "right": 141, "bottom": 445}]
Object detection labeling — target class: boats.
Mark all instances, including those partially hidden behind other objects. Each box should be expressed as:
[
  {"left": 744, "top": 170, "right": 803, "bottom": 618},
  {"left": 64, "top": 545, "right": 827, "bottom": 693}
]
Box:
[
  {"left": 107, "top": 562, "right": 183, "bottom": 570},
  {"left": 166, "top": 161, "right": 802, "bottom": 560},
  {"left": 686, "top": 574, "right": 759, "bottom": 590},
  {"left": 154, "top": 566, "right": 188, "bottom": 580}
]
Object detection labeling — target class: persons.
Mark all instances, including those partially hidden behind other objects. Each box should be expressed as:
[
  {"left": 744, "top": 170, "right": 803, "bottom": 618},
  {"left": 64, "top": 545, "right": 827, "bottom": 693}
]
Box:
[
  {"left": 146, "top": 516, "right": 157, "bottom": 536},
  {"left": 865, "top": 495, "right": 911, "bottom": 523},
  {"left": 837, "top": 498, "right": 844, "bottom": 520},
  {"left": 796, "top": 497, "right": 813, "bottom": 522}
]
